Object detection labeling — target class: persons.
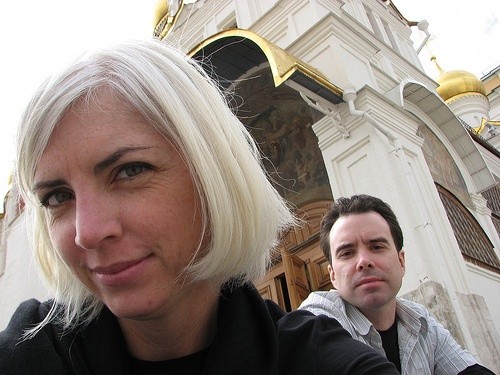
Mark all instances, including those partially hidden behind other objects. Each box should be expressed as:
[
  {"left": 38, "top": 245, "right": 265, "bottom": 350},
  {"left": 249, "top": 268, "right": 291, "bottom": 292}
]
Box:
[
  {"left": 298, "top": 193, "right": 496, "bottom": 375},
  {"left": 0, "top": 41, "right": 402, "bottom": 375}
]
